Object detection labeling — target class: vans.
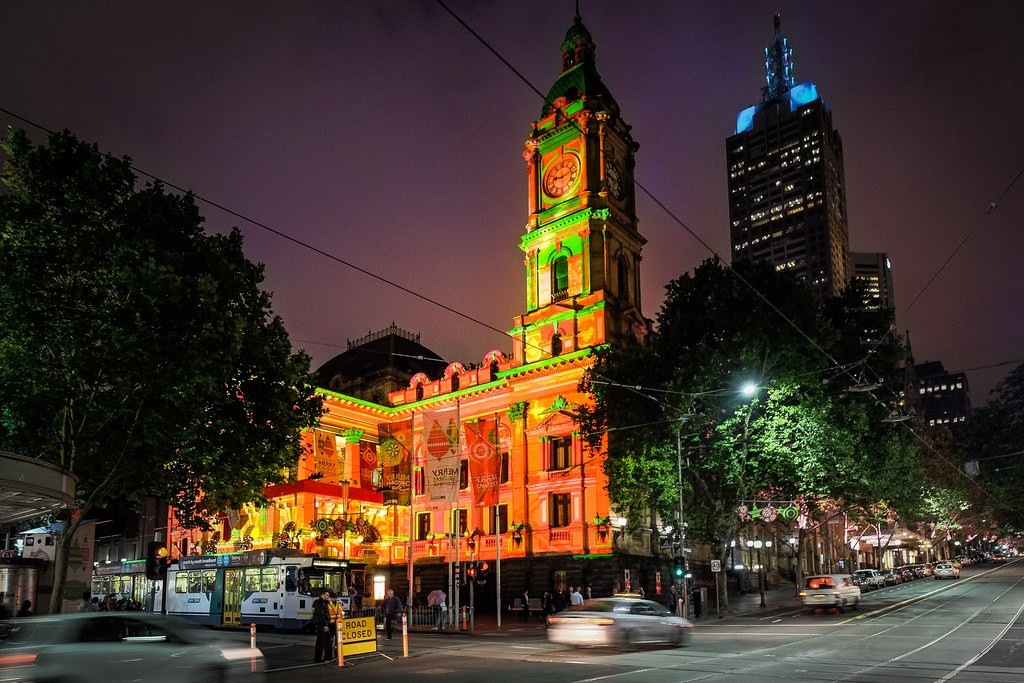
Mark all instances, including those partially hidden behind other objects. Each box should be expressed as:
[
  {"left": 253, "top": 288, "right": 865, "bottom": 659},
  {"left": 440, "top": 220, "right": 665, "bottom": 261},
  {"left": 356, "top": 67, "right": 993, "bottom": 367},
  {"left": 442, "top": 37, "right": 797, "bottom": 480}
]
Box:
[{"left": 801, "top": 573, "right": 860, "bottom": 614}]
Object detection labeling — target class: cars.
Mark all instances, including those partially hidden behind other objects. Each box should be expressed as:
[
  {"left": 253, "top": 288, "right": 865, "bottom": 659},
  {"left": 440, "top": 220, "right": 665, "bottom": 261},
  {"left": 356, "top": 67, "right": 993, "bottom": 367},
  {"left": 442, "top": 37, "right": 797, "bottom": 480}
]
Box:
[
  {"left": 547, "top": 597, "right": 694, "bottom": 648},
  {"left": 993, "top": 555, "right": 1006, "bottom": 564},
  {"left": 934, "top": 564, "right": 960, "bottom": 579},
  {"left": 851, "top": 550, "right": 1014, "bottom": 593}
]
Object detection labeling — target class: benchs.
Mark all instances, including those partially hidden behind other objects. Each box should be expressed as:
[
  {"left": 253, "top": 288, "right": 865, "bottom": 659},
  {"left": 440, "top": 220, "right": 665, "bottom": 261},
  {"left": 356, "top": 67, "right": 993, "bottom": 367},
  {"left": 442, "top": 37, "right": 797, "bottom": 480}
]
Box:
[{"left": 508, "top": 599, "right": 555, "bottom": 616}]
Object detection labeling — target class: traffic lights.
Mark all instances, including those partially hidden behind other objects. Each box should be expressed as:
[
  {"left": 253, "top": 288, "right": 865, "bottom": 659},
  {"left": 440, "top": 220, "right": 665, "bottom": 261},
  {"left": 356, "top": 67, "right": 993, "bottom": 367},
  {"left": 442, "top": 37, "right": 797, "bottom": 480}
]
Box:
[
  {"left": 468, "top": 568, "right": 475, "bottom": 583},
  {"left": 478, "top": 561, "right": 488, "bottom": 584},
  {"left": 149, "top": 542, "right": 167, "bottom": 580},
  {"left": 674, "top": 556, "right": 685, "bottom": 579}
]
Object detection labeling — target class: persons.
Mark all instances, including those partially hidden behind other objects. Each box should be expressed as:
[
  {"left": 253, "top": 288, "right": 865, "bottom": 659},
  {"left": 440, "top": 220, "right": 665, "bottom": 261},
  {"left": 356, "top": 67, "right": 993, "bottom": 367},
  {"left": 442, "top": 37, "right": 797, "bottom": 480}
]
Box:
[
  {"left": 521, "top": 587, "right": 593, "bottom": 625},
  {"left": 611, "top": 585, "right": 701, "bottom": 618},
  {"left": 0, "top": 581, "right": 143, "bottom": 643},
  {"left": 191, "top": 572, "right": 278, "bottom": 593},
  {"left": 288, "top": 577, "right": 447, "bottom": 663}
]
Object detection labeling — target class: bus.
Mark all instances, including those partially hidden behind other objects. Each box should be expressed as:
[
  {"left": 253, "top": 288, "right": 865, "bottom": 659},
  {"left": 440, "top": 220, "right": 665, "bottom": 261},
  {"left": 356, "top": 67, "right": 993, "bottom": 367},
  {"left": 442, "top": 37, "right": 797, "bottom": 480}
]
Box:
[{"left": 154, "top": 548, "right": 352, "bottom": 632}]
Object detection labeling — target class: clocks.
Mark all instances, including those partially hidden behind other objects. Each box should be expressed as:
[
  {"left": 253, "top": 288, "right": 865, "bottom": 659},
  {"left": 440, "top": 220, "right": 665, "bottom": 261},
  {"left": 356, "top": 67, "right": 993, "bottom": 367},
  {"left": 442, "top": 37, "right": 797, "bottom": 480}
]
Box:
[{"left": 544, "top": 153, "right": 580, "bottom": 199}]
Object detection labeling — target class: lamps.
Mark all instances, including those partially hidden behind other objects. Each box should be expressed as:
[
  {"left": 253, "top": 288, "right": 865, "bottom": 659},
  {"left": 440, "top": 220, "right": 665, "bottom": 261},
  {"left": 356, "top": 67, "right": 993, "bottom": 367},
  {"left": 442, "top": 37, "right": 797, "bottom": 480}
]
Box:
[
  {"left": 426, "top": 531, "right": 436, "bottom": 550},
  {"left": 510, "top": 522, "right": 522, "bottom": 546},
  {"left": 593, "top": 512, "right": 606, "bottom": 540}
]
